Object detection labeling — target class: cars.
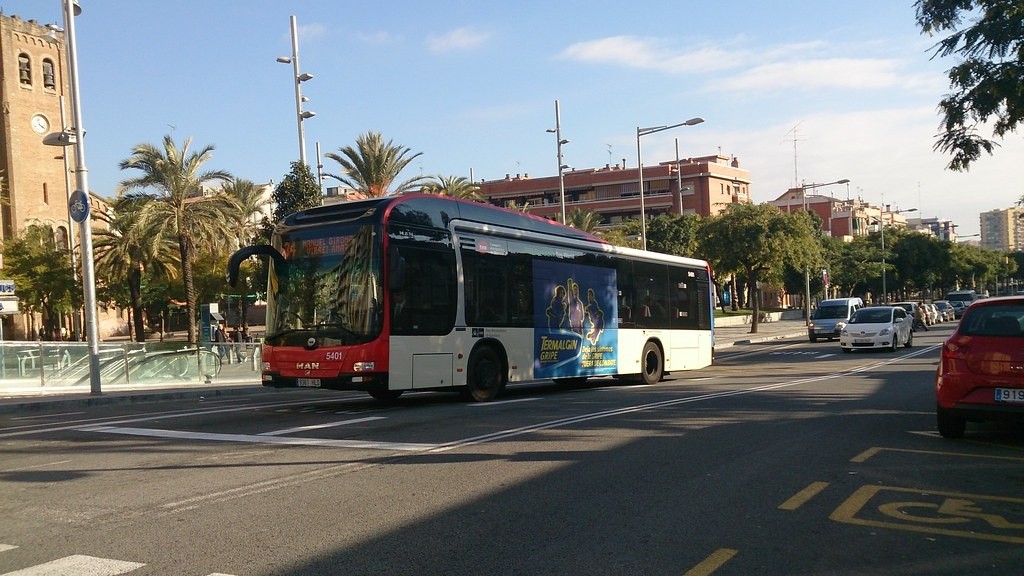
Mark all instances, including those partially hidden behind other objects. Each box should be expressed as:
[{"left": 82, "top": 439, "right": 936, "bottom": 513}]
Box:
[
  {"left": 840, "top": 307, "right": 913, "bottom": 352},
  {"left": 935, "top": 298, "right": 1024, "bottom": 439},
  {"left": 888, "top": 288, "right": 989, "bottom": 327}
]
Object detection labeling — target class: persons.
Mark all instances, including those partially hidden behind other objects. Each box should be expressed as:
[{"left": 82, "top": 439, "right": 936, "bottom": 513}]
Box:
[
  {"left": 61, "top": 325, "right": 66, "bottom": 340},
  {"left": 39, "top": 326, "right": 45, "bottom": 340},
  {"left": 215, "top": 324, "right": 253, "bottom": 365},
  {"left": 914, "top": 302, "right": 931, "bottom": 331},
  {"left": 373, "top": 286, "right": 415, "bottom": 329}
]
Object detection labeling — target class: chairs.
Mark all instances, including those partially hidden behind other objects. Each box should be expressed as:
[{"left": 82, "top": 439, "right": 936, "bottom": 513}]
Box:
[
  {"left": 619, "top": 304, "right": 678, "bottom": 319},
  {"left": 987, "top": 316, "right": 1023, "bottom": 333}
]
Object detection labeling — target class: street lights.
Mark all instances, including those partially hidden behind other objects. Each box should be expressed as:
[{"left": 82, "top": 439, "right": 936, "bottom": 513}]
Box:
[
  {"left": 879, "top": 208, "right": 919, "bottom": 305},
  {"left": 802, "top": 178, "right": 851, "bottom": 325},
  {"left": 954, "top": 233, "right": 980, "bottom": 243},
  {"left": 40, "top": 0, "right": 104, "bottom": 394},
  {"left": 317, "top": 165, "right": 324, "bottom": 205},
  {"left": 546, "top": 128, "right": 571, "bottom": 225},
  {"left": 637, "top": 117, "right": 705, "bottom": 252},
  {"left": 671, "top": 169, "right": 690, "bottom": 215},
  {"left": 276, "top": 57, "right": 316, "bottom": 169}
]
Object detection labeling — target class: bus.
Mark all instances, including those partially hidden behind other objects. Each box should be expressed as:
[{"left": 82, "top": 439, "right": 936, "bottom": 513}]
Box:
[{"left": 227, "top": 193, "right": 715, "bottom": 404}]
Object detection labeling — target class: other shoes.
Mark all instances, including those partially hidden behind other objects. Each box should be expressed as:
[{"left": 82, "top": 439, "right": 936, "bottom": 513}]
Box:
[
  {"left": 235, "top": 361, "right": 241, "bottom": 365},
  {"left": 244, "top": 357, "right": 247, "bottom": 362}
]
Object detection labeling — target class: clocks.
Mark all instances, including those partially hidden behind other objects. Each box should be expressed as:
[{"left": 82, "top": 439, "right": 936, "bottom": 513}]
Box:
[{"left": 33, "top": 115, "right": 47, "bottom": 133}]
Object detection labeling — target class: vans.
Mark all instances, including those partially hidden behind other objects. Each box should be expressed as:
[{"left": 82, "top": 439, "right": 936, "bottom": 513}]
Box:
[{"left": 807, "top": 297, "right": 865, "bottom": 343}]
